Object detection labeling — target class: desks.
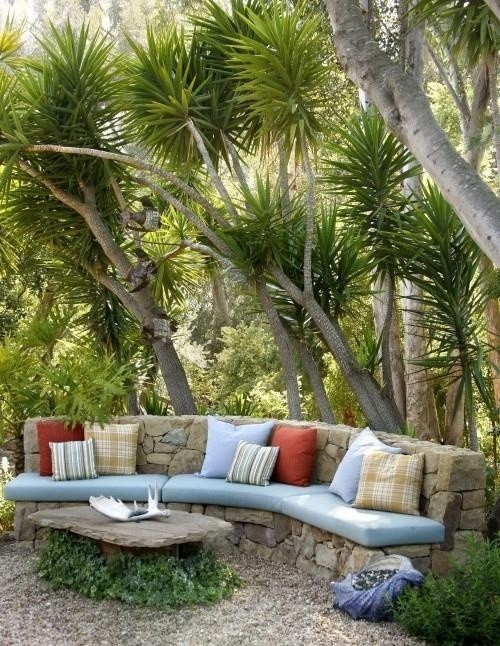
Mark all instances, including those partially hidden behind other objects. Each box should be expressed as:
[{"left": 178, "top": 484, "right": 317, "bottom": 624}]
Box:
[{"left": 26, "top": 506, "right": 235, "bottom": 561}]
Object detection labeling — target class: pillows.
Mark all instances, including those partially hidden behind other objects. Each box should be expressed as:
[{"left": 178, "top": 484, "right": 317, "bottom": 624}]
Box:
[
  {"left": 328, "top": 427, "right": 425, "bottom": 515},
  {"left": 197, "top": 416, "right": 319, "bottom": 487},
  {"left": 36, "top": 421, "right": 140, "bottom": 481}
]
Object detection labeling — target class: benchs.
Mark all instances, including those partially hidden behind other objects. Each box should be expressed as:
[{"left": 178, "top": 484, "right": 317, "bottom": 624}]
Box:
[{"left": 1, "top": 416, "right": 489, "bottom": 604}]
